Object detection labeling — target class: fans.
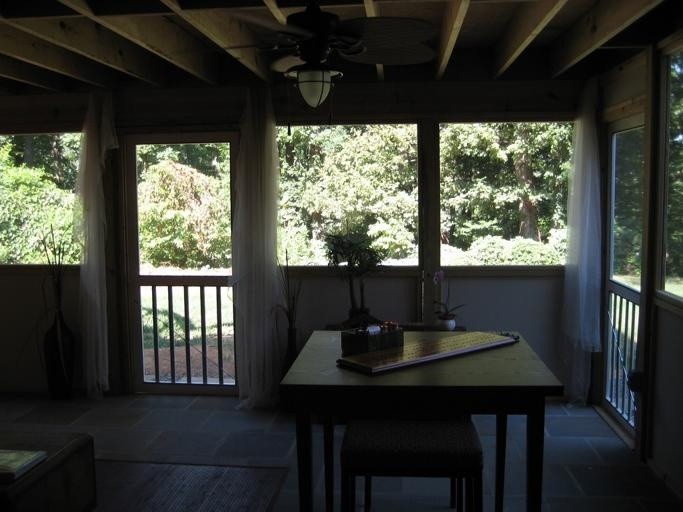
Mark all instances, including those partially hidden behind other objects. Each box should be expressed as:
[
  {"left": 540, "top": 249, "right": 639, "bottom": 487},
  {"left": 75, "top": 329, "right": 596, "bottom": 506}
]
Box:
[{"left": 215, "top": 2, "right": 440, "bottom": 66}]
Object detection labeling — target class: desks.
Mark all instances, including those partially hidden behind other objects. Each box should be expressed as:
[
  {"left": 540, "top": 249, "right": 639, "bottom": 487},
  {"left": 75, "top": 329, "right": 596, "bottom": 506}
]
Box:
[
  {"left": 279, "top": 330, "right": 565, "bottom": 512},
  {"left": 0, "top": 429, "right": 97, "bottom": 512}
]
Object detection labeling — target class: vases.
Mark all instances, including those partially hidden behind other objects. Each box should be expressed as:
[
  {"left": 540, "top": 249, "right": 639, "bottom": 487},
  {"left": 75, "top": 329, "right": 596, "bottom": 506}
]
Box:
[{"left": 433, "top": 318, "right": 456, "bottom": 330}]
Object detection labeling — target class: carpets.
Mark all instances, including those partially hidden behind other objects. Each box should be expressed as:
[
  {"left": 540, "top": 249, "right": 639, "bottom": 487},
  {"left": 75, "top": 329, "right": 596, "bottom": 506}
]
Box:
[{"left": 88, "top": 459, "right": 290, "bottom": 512}]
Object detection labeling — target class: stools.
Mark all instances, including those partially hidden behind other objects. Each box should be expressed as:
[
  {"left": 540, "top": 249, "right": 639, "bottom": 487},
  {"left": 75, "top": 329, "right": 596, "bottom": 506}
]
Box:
[{"left": 340, "top": 416, "right": 484, "bottom": 512}]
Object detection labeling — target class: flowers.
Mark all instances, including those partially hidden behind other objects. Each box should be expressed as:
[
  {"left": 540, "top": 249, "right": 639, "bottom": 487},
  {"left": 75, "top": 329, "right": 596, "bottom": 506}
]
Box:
[{"left": 432, "top": 270, "right": 465, "bottom": 319}]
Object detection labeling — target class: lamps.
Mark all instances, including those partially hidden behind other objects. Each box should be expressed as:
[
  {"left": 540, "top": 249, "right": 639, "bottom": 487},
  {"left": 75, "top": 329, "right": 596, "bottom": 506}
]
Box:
[{"left": 282, "top": 44, "right": 344, "bottom": 108}]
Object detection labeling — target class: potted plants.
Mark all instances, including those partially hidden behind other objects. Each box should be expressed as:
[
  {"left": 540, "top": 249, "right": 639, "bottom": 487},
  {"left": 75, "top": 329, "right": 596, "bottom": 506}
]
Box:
[
  {"left": 18, "top": 225, "right": 75, "bottom": 400},
  {"left": 271, "top": 250, "right": 303, "bottom": 416},
  {"left": 323, "top": 213, "right": 392, "bottom": 330}
]
